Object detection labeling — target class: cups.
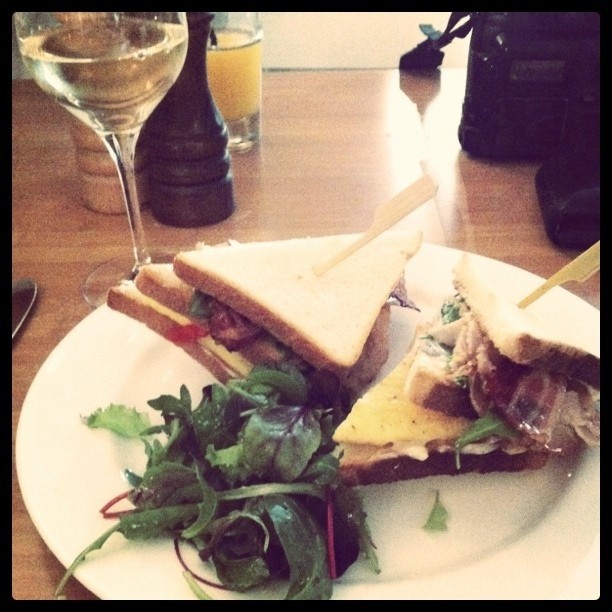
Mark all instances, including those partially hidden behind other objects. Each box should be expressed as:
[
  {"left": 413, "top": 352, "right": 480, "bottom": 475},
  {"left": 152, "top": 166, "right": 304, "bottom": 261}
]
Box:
[{"left": 202, "top": 13, "right": 261, "bottom": 150}]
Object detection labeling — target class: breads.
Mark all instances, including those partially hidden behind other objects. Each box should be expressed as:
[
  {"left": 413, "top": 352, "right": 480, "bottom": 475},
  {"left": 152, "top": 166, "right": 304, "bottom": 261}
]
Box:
[
  {"left": 172, "top": 228, "right": 424, "bottom": 379},
  {"left": 453, "top": 261, "right": 600, "bottom": 393},
  {"left": 135, "top": 264, "right": 388, "bottom": 384},
  {"left": 334, "top": 339, "right": 477, "bottom": 467},
  {"left": 108, "top": 283, "right": 254, "bottom": 386}
]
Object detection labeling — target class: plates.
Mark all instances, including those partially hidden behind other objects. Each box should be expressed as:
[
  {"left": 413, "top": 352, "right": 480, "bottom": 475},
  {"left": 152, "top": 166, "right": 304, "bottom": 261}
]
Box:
[{"left": 16, "top": 232, "right": 601, "bottom": 601}]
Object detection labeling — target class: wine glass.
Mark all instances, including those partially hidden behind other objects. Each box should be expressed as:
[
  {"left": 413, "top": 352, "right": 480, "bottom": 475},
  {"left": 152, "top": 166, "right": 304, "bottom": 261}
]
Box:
[{"left": 12, "top": 12, "right": 191, "bottom": 309}]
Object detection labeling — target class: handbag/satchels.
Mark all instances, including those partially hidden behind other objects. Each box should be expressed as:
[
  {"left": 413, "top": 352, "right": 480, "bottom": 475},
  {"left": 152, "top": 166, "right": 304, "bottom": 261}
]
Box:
[{"left": 399, "top": 13, "right": 601, "bottom": 163}]
